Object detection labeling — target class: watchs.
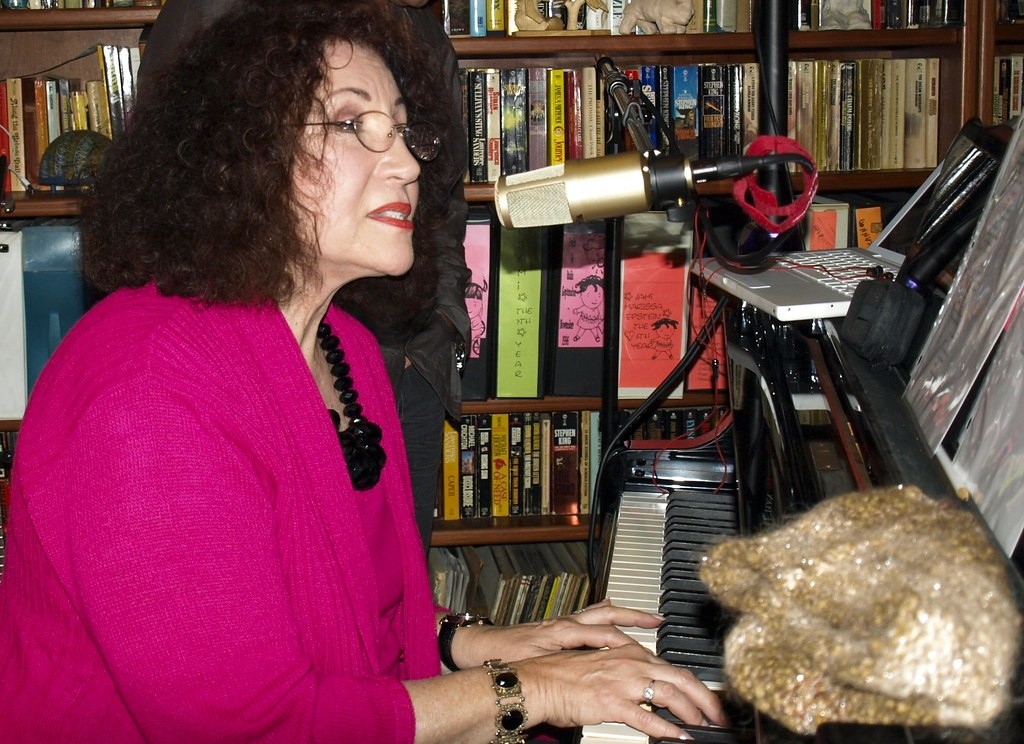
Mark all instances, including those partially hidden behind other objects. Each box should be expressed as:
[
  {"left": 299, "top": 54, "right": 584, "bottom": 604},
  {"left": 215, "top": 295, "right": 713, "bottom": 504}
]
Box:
[{"left": 438, "top": 611, "right": 494, "bottom": 672}]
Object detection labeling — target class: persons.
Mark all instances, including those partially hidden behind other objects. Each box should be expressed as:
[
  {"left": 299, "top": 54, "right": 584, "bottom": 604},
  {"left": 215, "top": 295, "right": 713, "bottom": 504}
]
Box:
[
  {"left": 138, "top": 0, "right": 471, "bottom": 563},
  {"left": 0, "top": 0, "right": 729, "bottom": 744}
]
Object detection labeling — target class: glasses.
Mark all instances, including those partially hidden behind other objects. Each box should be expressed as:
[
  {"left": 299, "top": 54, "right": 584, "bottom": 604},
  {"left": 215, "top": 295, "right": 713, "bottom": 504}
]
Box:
[{"left": 283, "top": 110, "right": 442, "bottom": 162}]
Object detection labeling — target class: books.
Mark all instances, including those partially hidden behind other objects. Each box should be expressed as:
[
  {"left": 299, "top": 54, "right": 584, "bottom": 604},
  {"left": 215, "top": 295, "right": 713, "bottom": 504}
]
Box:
[
  {"left": 465, "top": 190, "right": 882, "bottom": 402},
  {"left": 440, "top": 407, "right": 730, "bottom": 521},
  {"left": 0, "top": 0, "right": 166, "bottom": 484},
  {"left": 425, "top": 540, "right": 593, "bottom": 626},
  {"left": 442, "top": 0, "right": 1024, "bottom": 185}
]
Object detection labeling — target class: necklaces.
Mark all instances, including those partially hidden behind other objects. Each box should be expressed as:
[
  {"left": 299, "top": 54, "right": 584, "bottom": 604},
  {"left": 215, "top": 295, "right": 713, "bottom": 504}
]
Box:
[{"left": 304, "top": 325, "right": 385, "bottom": 491}]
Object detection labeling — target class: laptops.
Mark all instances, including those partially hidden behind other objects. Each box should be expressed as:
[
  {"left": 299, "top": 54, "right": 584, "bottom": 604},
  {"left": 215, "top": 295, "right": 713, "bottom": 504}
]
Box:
[{"left": 684, "top": 158, "right": 972, "bottom": 322}]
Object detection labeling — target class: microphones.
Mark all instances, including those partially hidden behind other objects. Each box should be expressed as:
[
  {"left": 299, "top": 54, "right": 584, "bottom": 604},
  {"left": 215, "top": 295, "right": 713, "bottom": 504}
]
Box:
[{"left": 494, "top": 148, "right": 752, "bottom": 229}]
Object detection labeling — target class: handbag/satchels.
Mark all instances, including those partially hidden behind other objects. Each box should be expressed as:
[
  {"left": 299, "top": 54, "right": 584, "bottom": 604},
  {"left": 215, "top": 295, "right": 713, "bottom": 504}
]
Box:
[{"left": 840, "top": 279, "right": 925, "bottom": 367}]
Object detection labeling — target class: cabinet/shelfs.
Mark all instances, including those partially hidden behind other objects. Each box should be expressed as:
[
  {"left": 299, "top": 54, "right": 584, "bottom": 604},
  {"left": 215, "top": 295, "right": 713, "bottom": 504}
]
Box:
[{"left": 0, "top": 0, "right": 1024, "bottom": 550}]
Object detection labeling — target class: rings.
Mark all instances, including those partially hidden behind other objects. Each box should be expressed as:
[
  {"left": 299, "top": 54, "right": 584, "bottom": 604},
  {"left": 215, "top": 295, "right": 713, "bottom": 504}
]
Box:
[
  {"left": 572, "top": 608, "right": 586, "bottom": 615},
  {"left": 643, "top": 679, "right": 655, "bottom": 707}
]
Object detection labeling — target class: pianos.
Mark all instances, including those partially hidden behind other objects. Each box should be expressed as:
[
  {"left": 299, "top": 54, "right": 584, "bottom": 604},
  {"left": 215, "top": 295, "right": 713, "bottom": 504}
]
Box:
[{"left": 572, "top": 115, "right": 1023, "bottom": 744}]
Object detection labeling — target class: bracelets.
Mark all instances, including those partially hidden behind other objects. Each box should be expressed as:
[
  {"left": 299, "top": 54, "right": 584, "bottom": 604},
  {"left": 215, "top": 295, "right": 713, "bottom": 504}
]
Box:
[{"left": 482, "top": 659, "right": 528, "bottom": 744}]
